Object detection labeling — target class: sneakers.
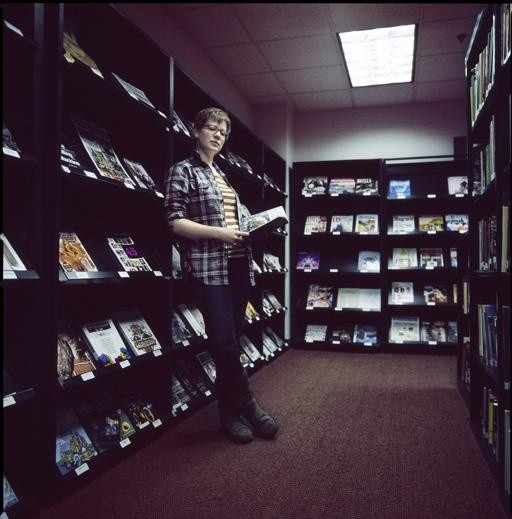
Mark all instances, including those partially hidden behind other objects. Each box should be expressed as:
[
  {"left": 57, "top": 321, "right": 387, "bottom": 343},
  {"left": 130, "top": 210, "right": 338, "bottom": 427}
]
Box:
[
  {"left": 240, "top": 395, "right": 278, "bottom": 440},
  {"left": 220, "top": 408, "right": 254, "bottom": 444}
]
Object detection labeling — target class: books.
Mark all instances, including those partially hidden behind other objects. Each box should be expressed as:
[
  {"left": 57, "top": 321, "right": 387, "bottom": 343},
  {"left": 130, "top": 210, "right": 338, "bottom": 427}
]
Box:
[
  {"left": 295, "top": 176, "right": 469, "bottom": 345},
  {"left": 1, "top": 234, "right": 28, "bottom": 271},
  {"left": 468, "top": 0, "right": 512, "bottom": 495},
  {"left": 2, "top": 129, "right": 22, "bottom": 152},
  {"left": 56, "top": 32, "right": 217, "bottom": 475},
  {"left": 239, "top": 253, "right": 289, "bottom": 367},
  {"left": 3, "top": 477, "right": 19, "bottom": 510},
  {"left": 219, "top": 149, "right": 288, "bottom": 196},
  {"left": 243, "top": 205, "right": 289, "bottom": 240}
]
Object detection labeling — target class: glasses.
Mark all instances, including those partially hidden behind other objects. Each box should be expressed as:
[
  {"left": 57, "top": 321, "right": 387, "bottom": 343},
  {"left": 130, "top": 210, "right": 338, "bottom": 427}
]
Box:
[{"left": 202, "top": 124, "right": 227, "bottom": 136}]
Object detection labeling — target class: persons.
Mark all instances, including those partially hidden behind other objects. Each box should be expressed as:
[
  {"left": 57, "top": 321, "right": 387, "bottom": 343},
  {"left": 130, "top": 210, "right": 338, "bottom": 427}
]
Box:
[{"left": 165, "top": 107, "right": 279, "bottom": 443}]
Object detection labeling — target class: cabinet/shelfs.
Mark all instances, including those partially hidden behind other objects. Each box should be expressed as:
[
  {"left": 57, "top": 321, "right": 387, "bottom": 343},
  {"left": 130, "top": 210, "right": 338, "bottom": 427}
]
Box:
[
  {"left": 0, "top": 2, "right": 286, "bottom": 514},
  {"left": 291, "top": 154, "right": 468, "bottom": 357},
  {"left": 459, "top": 0, "right": 511, "bottom": 516}
]
